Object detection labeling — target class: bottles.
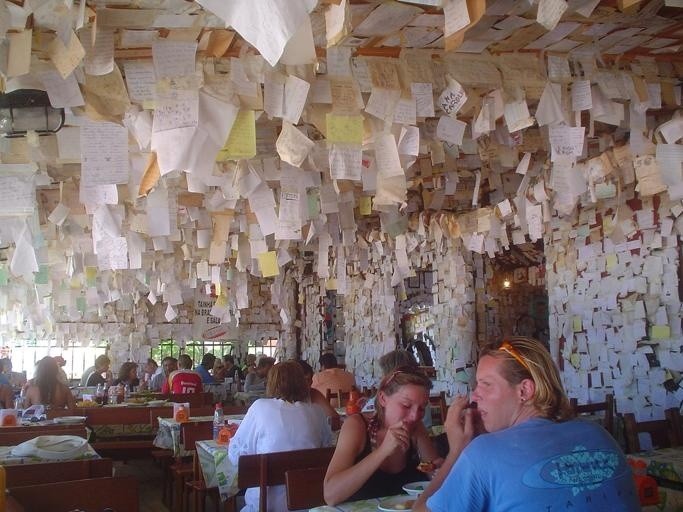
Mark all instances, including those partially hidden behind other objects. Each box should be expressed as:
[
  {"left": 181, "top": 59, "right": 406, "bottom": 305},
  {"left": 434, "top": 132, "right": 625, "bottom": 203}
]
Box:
[
  {"left": 346, "top": 386, "right": 376, "bottom": 415},
  {"left": 235, "top": 370, "right": 241, "bottom": 393},
  {"left": 15, "top": 395, "right": 22, "bottom": 424},
  {"left": 175, "top": 405, "right": 188, "bottom": 423},
  {"left": 97, "top": 382, "right": 130, "bottom": 405},
  {"left": 213, "top": 403, "right": 232, "bottom": 445}
]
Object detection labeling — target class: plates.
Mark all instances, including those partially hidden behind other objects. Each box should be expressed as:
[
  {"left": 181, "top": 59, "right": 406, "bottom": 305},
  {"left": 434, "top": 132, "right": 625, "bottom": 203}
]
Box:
[
  {"left": 77, "top": 386, "right": 96, "bottom": 390},
  {"left": 126, "top": 399, "right": 167, "bottom": 406},
  {"left": 11, "top": 436, "right": 88, "bottom": 460},
  {"left": 378, "top": 482, "right": 432, "bottom": 512},
  {"left": 206, "top": 380, "right": 224, "bottom": 385},
  {"left": 55, "top": 415, "right": 87, "bottom": 424}
]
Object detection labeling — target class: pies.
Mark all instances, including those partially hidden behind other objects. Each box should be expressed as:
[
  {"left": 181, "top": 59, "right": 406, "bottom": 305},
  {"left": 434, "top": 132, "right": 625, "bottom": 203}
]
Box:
[{"left": 418, "top": 461, "right": 435, "bottom": 474}]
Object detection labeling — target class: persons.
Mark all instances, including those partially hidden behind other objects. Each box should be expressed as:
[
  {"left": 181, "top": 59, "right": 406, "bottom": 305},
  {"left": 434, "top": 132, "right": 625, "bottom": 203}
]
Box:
[
  {"left": 411, "top": 336, "right": 641, "bottom": 510},
  {"left": 1, "top": 353, "right": 339, "bottom": 428},
  {"left": 356, "top": 351, "right": 433, "bottom": 433},
  {"left": 227, "top": 360, "right": 333, "bottom": 512},
  {"left": 323, "top": 366, "right": 445, "bottom": 506},
  {"left": 310, "top": 353, "right": 356, "bottom": 409}
]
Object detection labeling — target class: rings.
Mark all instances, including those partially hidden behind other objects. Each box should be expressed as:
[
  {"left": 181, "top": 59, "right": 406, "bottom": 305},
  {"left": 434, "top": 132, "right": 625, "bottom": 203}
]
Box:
[{"left": 399, "top": 434, "right": 402, "bottom": 440}]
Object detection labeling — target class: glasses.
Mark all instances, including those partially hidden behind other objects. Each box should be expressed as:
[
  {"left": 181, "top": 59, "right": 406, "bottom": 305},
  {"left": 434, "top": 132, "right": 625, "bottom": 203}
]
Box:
[
  {"left": 483, "top": 341, "right": 529, "bottom": 370},
  {"left": 381, "top": 369, "right": 425, "bottom": 391}
]
